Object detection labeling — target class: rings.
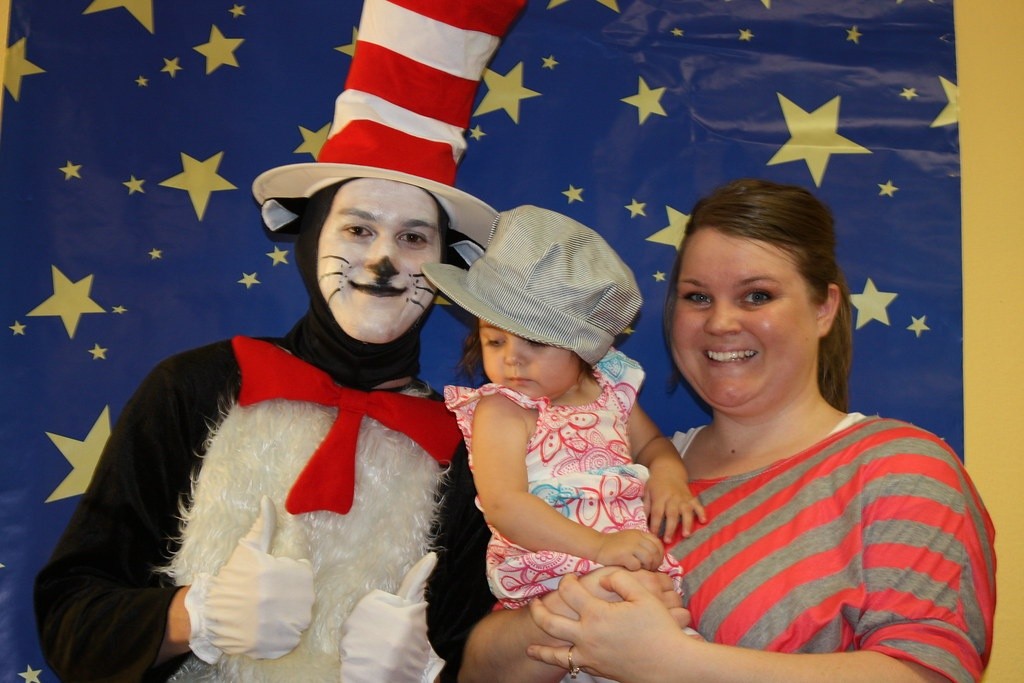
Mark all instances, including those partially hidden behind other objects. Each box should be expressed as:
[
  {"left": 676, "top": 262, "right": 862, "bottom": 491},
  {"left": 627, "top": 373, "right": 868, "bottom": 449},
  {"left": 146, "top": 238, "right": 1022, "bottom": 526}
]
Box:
[{"left": 568, "top": 646, "right": 579, "bottom": 678}]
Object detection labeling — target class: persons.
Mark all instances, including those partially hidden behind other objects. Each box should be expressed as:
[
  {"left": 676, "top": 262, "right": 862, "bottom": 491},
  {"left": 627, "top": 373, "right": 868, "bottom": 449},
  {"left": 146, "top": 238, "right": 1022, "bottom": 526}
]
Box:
[
  {"left": 469, "top": 205, "right": 707, "bottom": 683},
  {"left": 459, "top": 181, "right": 998, "bottom": 683},
  {"left": 32, "top": 175, "right": 495, "bottom": 683}
]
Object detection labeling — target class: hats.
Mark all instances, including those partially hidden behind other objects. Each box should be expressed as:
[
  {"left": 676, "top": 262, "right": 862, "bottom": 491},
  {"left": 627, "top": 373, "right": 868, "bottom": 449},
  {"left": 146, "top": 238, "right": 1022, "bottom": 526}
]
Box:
[
  {"left": 420, "top": 204, "right": 642, "bottom": 366},
  {"left": 252, "top": 0, "right": 526, "bottom": 250}
]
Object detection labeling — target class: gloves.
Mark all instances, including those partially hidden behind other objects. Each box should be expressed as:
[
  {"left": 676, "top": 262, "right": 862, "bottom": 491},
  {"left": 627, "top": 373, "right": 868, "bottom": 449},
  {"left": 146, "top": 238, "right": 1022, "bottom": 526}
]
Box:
[
  {"left": 338, "top": 551, "right": 447, "bottom": 683},
  {"left": 184, "top": 494, "right": 317, "bottom": 665}
]
